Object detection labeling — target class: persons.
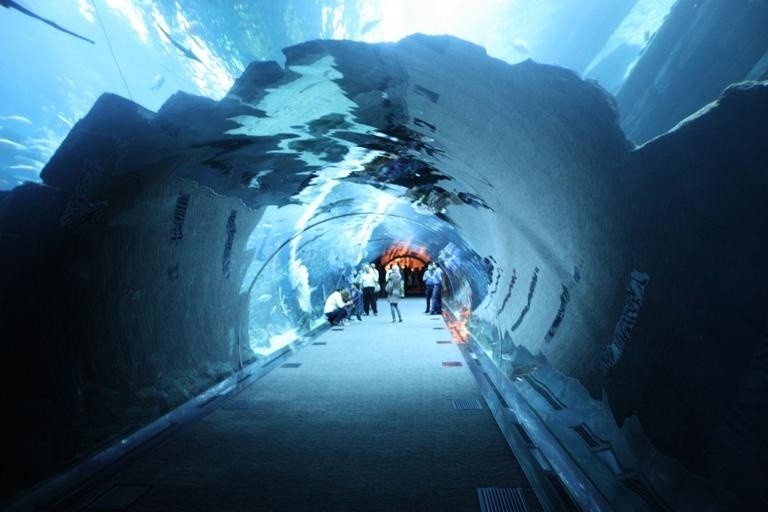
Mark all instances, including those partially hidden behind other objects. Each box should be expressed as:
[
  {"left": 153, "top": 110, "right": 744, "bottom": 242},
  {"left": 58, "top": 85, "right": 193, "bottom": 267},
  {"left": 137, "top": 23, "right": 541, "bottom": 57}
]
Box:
[{"left": 322, "top": 258, "right": 445, "bottom": 327}]
